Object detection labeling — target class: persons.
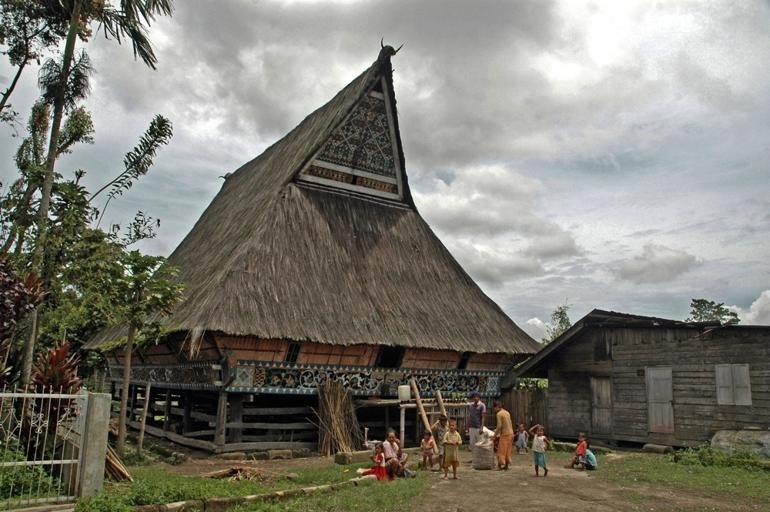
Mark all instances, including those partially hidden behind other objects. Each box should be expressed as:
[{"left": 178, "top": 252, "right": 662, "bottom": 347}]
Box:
[
  {"left": 492, "top": 400, "right": 514, "bottom": 471},
  {"left": 514, "top": 423, "right": 529, "bottom": 454},
  {"left": 528, "top": 424, "right": 551, "bottom": 477},
  {"left": 564, "top": 432, "right": 598, "bottom": 470},
  {"left": 467, "top": 391, "right": 486, "bottom": 450},
  {"left": 383, "top": 430, "right": 409, "bottom": 479},
  {"left": 420, "top": 415, "right": 462, "bottom": 480},
  {"left": 362, "top": 442, "right": 385, "bottom": 475}
]
